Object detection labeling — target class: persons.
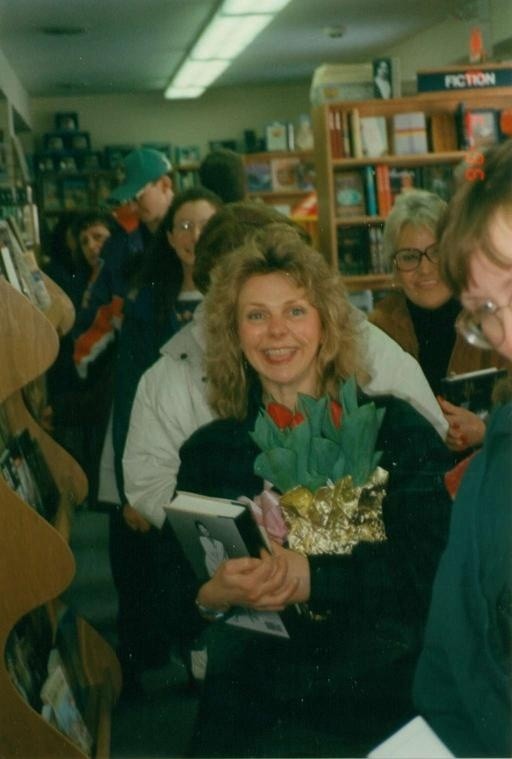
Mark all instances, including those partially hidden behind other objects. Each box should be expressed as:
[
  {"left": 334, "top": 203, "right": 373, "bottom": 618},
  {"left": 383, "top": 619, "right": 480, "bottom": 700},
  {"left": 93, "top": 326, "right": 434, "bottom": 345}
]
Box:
[
  {"left": 359, "top": 186, "right": 508, "bottom": 472},
  {"left": 406, "top": 137, "right": 510, "bottom": 756},
  {"left": 55, "top": 148, "right": 243, "bottom": 697},
  {"left": 123, "top": 204, "right": 451, "bottom": 529},
  {"left": 196, "top": 517, "right": 229, "bottom": 582},
  {"left": 149, "top": 218, "right": 452, "bottom": 754}
]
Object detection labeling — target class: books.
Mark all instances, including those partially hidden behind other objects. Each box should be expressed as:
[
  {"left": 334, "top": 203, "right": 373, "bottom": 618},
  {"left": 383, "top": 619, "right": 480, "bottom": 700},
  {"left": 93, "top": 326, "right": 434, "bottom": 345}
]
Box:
[
  {"left": 2, "top": 185, "right": 101, "bottom": 759},
  {"left": 327, "top": 107, "right": 460, "bottom": 275},
  {"left": 438, "top": 363, "right": 507, "bottom": 463},
  {"left": 163, "top": 490, "right": 291, "bottom": 637},
  {"left": 455, "top": 101, "right": 512, "bottom": 151},
  {"left": 249, "top": 123, "right": 319, "bottom": 218}
]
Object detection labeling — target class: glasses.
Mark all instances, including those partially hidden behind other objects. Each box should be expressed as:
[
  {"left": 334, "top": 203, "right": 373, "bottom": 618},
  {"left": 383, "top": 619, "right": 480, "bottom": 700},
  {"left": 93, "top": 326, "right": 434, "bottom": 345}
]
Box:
[
  {"left": 389, "top": 242, "right": 441, "bottom": 271},
  {"left": 454, "top": 300, "right": 512, "bottom": 350}
]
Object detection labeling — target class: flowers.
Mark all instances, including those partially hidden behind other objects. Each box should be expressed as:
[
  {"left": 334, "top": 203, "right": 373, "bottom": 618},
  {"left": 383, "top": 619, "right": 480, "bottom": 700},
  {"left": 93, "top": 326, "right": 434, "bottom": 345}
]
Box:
[{"left": 248, "top": 373, "right": 388, "bottom": 553}]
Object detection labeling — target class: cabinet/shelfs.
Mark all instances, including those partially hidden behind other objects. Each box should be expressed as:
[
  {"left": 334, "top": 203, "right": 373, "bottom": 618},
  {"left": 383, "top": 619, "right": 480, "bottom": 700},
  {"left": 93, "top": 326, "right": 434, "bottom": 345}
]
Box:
[
  {"left": 311, "top": 97, "right": 512, "bottom": 296},
  {"left": 241, "top": 150, "right": 320, "bottom": 237}
]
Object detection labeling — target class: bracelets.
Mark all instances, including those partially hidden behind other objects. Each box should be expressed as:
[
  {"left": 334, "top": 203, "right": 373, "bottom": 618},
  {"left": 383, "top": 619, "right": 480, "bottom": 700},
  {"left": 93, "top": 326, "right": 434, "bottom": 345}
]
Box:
[{"left": 192, "top": 599, "right": 228, "bottom": 626}]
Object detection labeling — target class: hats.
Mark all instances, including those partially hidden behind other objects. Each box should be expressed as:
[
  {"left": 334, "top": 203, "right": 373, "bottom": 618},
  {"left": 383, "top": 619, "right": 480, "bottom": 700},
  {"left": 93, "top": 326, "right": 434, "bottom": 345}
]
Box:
[{"left": 103, "top": 148, "right": 172, "bottom": 208}]
